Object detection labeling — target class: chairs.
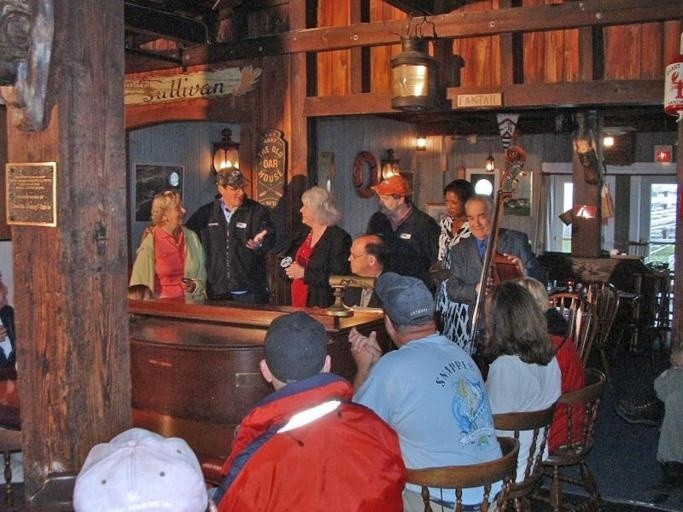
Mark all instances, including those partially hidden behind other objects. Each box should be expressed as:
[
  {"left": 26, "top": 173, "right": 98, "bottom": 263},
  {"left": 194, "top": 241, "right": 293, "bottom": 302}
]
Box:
[{"left": 0, "top": 429, "right": 26, "bottom": 512}]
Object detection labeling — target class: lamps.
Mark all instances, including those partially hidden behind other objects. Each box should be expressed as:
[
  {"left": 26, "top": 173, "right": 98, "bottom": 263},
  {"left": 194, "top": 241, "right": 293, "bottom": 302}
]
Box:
[
  {"left": 386, "top": 10, "right": 452, "bottom": 119},
  {"left": 210, "top": 129, "right": 239, "bottom": 173},
  {"left": 381, "top": 147, "right": 400, "bottom": 182},
  {"left": 485, "top": 152, "right": 496, "bottom": 172},
  {"left": 414, "top": 132, "right": 427, "bottom": 150}
]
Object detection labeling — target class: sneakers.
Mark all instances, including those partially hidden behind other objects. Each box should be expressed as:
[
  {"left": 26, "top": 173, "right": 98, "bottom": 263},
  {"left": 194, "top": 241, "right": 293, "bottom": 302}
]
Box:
[{"left": 614, "top": 398, "right": 664, "bottom": 426}]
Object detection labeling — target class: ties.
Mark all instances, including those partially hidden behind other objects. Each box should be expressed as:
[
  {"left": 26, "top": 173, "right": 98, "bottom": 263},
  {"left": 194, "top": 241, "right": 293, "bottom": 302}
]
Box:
[{"left": 482, "top": 240, "right": 489, "bottom": 262}]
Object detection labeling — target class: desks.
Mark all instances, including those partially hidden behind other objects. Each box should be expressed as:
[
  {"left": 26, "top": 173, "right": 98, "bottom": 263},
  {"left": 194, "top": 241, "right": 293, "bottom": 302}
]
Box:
[{"left": 118, "top": 298, "right": 386, "bottom": 427}]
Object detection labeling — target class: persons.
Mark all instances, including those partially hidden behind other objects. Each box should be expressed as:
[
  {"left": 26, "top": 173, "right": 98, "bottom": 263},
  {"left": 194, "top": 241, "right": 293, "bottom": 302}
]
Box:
[
  {"left": 483, "top": 280, "right": 564, "bottom": 485},
  {"left": 126, "top": 191, "right": 208, "bottom": 305},
  {"left": 284, "top": 176, "right": 547, "bottom": 355},
  {"left": 207, "top": 311, "right": 406, "bottom": 512},
  {"left": 0, "top": 274, "right": 21, "bottom": 431},
  {"left": 348, "top": 271, "right": 507, "bottom": 512},
  {"left": 518, "top": 276, "right": 587, "bottom": 455},
  {"left": 611, "top": 340, "right": 683, "bottom": 501},
  {"left": 141, "top": 167, "right": 276, "bottom": 305}
]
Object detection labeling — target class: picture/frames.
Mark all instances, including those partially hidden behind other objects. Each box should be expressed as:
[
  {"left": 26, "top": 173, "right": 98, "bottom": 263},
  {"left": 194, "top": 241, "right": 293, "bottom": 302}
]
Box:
[{"left": 464, "top": 167, "right": 500, "bottom": 203}]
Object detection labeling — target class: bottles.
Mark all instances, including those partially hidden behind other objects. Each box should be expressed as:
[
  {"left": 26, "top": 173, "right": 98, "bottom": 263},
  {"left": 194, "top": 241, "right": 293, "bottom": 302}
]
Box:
[{"left": 566, "top": 276, "right": 576, "bottom": 293}]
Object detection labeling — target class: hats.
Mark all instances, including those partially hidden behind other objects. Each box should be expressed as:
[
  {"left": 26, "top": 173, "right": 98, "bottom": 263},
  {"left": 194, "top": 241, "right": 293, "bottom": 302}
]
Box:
[
  {"left": 216, "top": 168, "right": 250, "bottom": 187},
  {"left": 264, "top": 311, "right": 328, "bottom": 384},
  {"left": 370, "top": 175, "right": 410, "bottom": 197},
  {"left": 375, "top": 273, "right": 434, "bottom": 327}
]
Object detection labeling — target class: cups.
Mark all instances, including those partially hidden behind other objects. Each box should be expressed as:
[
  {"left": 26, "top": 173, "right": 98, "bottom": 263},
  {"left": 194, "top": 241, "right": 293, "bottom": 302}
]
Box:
[{"left": 280, "top": 256, "right": 294, "bottom": 270}]
